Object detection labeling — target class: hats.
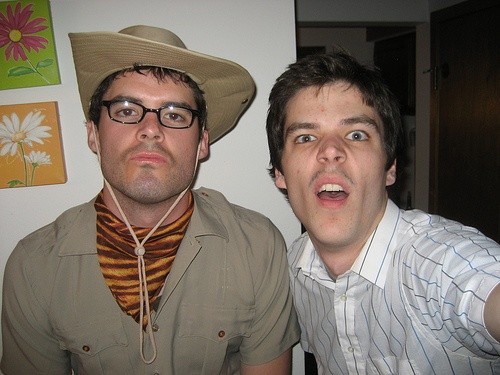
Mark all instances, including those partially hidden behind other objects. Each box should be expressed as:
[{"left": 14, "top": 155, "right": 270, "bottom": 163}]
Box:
[{"left": 68, "top": 25, "right": 257, "bottom": 146}]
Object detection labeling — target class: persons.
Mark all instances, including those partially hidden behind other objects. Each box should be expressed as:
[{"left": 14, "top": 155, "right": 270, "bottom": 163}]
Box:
[
  {"left": 0, "top": 25, "right": 302, "bottom": 375},
  {"left": 265, "top": 50, "right": 500, "bottom": 375}
]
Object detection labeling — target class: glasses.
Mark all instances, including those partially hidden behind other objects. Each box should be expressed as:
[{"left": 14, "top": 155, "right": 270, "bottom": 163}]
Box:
[{"left": 95, "top": 100, "right": 204, "bottom": 129}]
